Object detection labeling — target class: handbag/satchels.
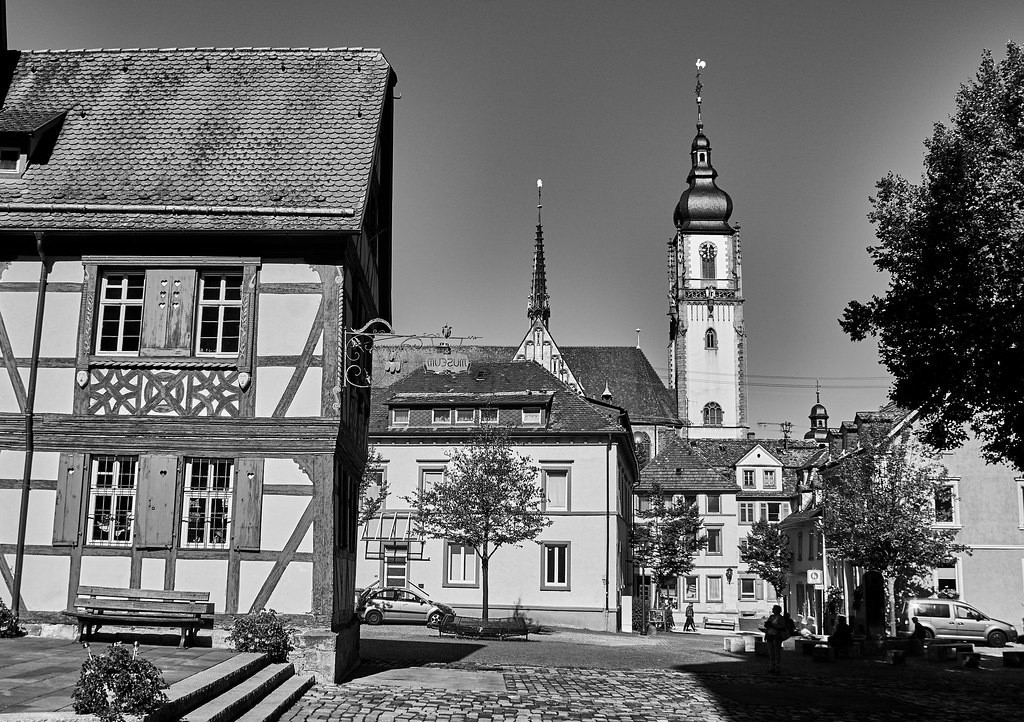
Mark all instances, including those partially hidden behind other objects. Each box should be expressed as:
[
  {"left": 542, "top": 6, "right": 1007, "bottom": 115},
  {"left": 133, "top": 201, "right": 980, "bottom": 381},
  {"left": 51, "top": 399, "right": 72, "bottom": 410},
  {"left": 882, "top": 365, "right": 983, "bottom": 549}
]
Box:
[{"left": 779, "top": 616, "right": 791, "bottom": 641}]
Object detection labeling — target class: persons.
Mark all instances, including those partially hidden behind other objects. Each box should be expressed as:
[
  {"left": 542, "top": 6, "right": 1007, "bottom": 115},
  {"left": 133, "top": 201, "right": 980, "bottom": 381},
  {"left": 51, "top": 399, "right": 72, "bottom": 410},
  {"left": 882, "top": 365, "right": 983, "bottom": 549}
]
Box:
[
  {"left": 827, "top": 615, "right": 851, "bottom": 662},
  {"left": 764, "top": 605, "right": 816, "bottom": 662},
  {"left": 682, "top": 603, "right": 699, "bottom": 631},
  {"left": 910, "top": 617, "right": 926, "bottom": 652}
]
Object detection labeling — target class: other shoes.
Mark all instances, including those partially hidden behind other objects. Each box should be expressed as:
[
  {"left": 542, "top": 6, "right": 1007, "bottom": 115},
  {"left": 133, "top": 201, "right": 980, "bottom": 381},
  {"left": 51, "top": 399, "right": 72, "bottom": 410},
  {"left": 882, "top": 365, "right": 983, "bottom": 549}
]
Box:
[
  {"left": 694, "top": 629, "right": 698, "bottom": 632},
  {"left": 686, "top": 629, "right": 691, "bottom": 631},
  {"left": 683, "top": 629, "right": 686, "bottom": 631},
  {"left": 766, "top": 670, "right": 780, "bottom": 676}
]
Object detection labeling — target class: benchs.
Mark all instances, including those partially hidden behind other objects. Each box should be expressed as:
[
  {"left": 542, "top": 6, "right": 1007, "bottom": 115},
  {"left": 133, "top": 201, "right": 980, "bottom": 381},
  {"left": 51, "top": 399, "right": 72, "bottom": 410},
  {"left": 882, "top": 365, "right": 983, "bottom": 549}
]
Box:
[
  {"left": 793, "top": 638, "right": 1024, "bottom": 667},
  {"left": 67, "top": 590, "right": 216, "bottom": 650},
  {"left": 703, "top": 617, "right": 735, "bottom": 630}
]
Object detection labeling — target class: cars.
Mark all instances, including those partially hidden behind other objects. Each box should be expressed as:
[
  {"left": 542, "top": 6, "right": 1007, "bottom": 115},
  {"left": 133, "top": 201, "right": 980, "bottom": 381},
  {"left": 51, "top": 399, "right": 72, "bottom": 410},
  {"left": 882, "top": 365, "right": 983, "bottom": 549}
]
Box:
[
  {"left": 354, "top": 587, "right": 456, "bottom": 627},
  {"left": 896, "top": 598, "right": 1020, "bottom": 648}
]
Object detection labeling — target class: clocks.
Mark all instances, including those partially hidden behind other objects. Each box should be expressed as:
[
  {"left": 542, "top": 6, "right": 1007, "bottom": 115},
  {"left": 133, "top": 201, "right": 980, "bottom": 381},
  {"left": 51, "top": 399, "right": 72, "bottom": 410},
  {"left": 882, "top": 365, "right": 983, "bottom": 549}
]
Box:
[{"left": 699, "top": 241, "right": 718, "bottom": 259}]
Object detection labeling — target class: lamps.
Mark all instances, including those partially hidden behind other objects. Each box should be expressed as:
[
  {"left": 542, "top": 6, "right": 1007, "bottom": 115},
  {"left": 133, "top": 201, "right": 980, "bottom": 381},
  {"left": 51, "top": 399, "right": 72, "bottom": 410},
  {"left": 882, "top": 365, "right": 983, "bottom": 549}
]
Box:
[{"left": 727, "top": 567, "right": 733, "bottom": 584}]
